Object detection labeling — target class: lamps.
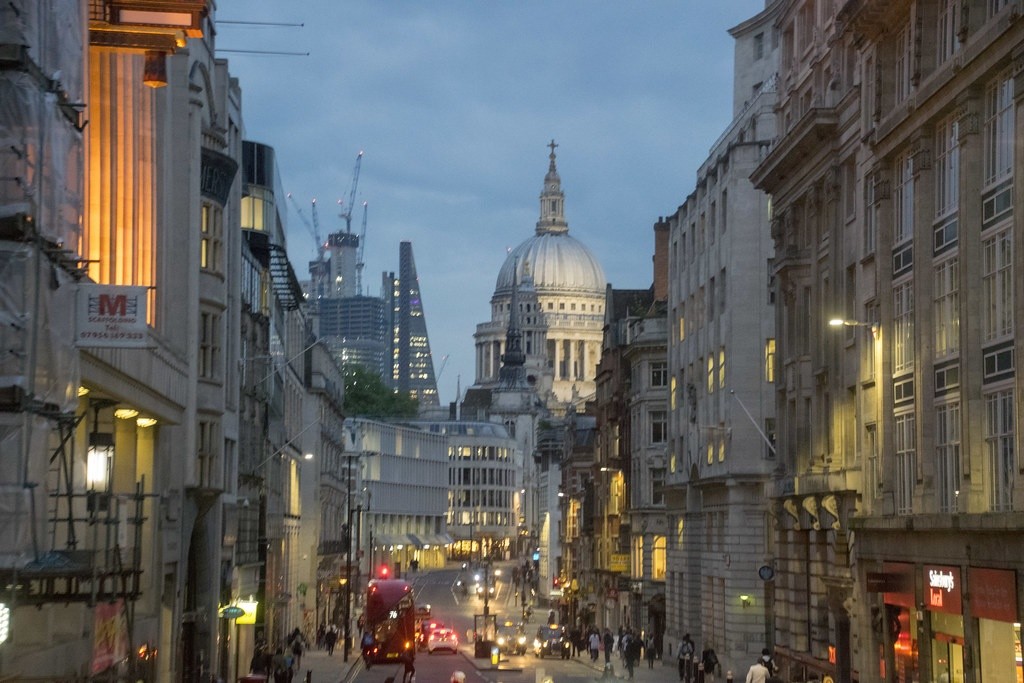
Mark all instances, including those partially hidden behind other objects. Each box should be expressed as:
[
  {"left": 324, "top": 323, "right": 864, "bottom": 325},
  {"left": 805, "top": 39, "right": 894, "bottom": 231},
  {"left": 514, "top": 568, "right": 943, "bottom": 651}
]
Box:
[
  {"left": 738, "top": 593, "right": 750, "bottom": 608},
  {"left": 828, "top": 318, "right": 880, "bottom": 339},
  {"left": 85, "top": 399, "right": 121, "bottom": 511},
  {"left": 114, "top": 403, "right": 141, "bottom": 419},
  {"left": 65, "top": 382, "right": 89, "bottom": 398},
  {"left": 135, "top": 413, "right": 158, "bottom": 427}
]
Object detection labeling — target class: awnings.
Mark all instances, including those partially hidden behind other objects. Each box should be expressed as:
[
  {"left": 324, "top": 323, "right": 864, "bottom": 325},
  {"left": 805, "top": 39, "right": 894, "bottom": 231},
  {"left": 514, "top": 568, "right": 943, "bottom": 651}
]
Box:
[{"left": 372, "top": 533, "right": 454, "bottom": 547}]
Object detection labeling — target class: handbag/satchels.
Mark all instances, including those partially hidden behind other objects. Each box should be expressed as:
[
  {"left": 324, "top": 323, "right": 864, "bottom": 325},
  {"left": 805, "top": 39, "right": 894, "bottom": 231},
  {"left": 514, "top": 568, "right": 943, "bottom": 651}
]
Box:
[{"left": 707, "top": 650, "right": 718, "bottom": 664}]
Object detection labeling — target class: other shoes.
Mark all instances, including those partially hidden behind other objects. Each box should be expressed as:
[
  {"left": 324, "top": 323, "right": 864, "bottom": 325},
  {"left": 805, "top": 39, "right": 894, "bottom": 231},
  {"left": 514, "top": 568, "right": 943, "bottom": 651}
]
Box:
[
  {"left": 591, "top": 655, "right": 593, "bottom": 659},
  {"left": 593, "top": 658, "right": 597, "bottom": 662},
  {"left": 572, "top": 654, "right": 575, "bottom": 657},
  {"left": 680, "top": 677, "right": 683, "bottom": 681},
  {"left": 329, "top": 652, "right": 333, "bottom": 655},
  {"left": 629, "top": 674, "right": 633, "bottom": 678},
  {"left": 578, "top": 655, "right": 580, "bottom": 658},
  {"left": 638, "top": 663, "right": 640, "bottom": 667},
  {"left": 651, "top": 667, "right": 653, "bottom": 669},
  {"left": 648, "top": 667, "right": 650, "bottom": 669}
]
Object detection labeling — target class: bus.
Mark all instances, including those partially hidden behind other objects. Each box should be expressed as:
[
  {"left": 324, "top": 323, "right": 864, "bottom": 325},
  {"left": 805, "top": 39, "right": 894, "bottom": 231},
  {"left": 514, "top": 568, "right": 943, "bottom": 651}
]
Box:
[{"left": 360, "top": 579, "right": 418, "bottom": 668}]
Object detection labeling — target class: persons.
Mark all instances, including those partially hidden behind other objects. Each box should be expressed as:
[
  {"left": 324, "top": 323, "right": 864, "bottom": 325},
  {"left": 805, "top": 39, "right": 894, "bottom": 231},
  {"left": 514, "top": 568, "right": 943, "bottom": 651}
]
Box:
[
  {"left": 547, "top": 608, "right": 658, "bottom": 678},
  {"left": 676, "top": 633, "right": 719, "bottom": 683},
  {"left": 249, "top": 610, "right": 374, "bottom": 683},
  {"left": 400, "top": 648, "right": 415, "bottom": 683},
  {"left": 746, "top": 648, "right": 778, "bottom": 683},
  {"left": 512, "top": 560, "right": 539, "bottom": 586}
]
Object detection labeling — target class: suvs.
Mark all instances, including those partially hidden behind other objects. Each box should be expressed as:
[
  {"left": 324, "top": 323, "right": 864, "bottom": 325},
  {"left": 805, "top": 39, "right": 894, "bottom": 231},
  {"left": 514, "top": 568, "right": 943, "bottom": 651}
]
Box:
[{"left": 534, "top": 624, "right": 571, "bottom": 661}]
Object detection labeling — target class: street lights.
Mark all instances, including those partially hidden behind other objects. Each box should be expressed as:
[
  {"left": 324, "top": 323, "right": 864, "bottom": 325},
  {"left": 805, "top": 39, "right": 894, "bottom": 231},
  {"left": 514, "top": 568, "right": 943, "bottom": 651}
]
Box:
[{"left": 334, "top": 414, "right": 363, "bottom": 661}]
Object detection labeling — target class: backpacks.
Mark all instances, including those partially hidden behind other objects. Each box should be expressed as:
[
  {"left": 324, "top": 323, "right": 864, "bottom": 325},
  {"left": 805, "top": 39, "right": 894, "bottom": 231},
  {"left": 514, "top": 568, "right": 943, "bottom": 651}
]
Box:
[
  {"left": 647, "top": 637, "right": 655, "bottom": 649},
  {"left": 681, "top": 642, "right": 689, "bottom": 655},
  {"left": 294, "top": 642, "right": 301, "bottom": 656},
  {"left": 762, "top": 657, "right": 773, "bottom": 677}
]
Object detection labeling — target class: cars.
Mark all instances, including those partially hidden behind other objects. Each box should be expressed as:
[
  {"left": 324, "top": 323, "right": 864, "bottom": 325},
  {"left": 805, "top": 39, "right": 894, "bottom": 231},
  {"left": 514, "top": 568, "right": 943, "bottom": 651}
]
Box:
[
  {"left": 414, "top": 602, "right": 446, "bottom": 651},
  {"left": 496, "top": 627, "right": 528, "bottom": 657},
  {"left": 427, "top": 628, "right": 458, "bottom": 655}
]
